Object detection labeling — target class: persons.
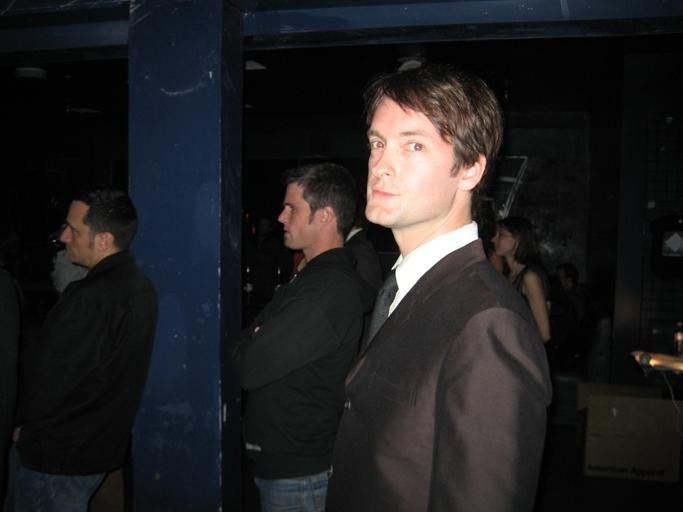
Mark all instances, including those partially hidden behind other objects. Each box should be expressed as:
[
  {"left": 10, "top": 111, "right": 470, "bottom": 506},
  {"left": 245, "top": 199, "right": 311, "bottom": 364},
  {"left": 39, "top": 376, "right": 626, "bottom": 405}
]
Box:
[
  {"left": 326, "top": 54, "right": 552, "bottom": 510},
  {"left": 10, "top": 182, "right": 159, "bottom": 512},
  {"left": 232, "top": 155, "right": 362, "bottom": 512},
  {"left": 344, "top": 202, "right": 384, "bottom": 313},
  {"left": 487, "top": 214, "right": 553, "bottom": 344}
]
